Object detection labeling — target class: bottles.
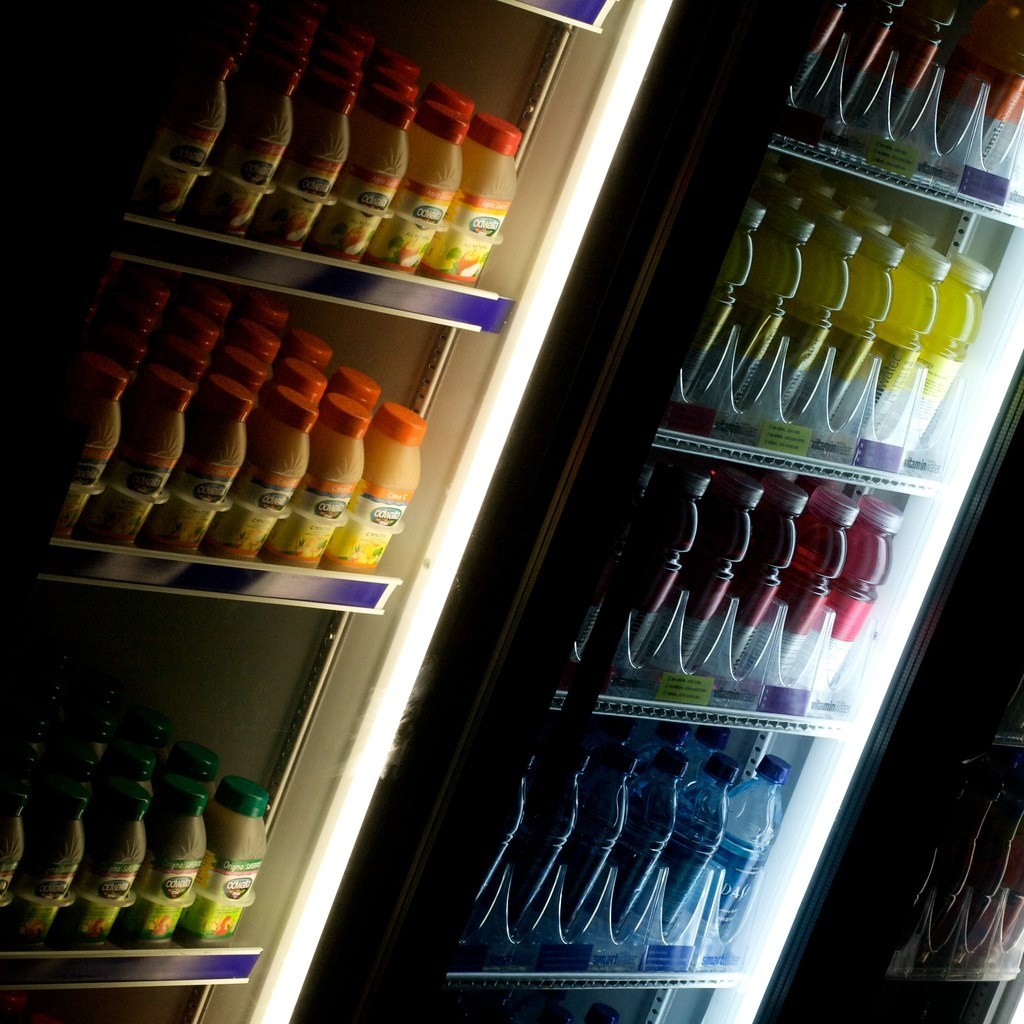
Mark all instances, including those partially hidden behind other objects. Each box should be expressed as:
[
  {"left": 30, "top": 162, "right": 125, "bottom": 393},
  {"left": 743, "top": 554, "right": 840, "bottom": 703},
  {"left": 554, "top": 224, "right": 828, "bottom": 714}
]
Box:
[
  {"left": 0, "top": 25, "right": 522, "bottom": 947},
  {"left": 399, "top": 0, "right": 1024, "bottom": 1024}
]
